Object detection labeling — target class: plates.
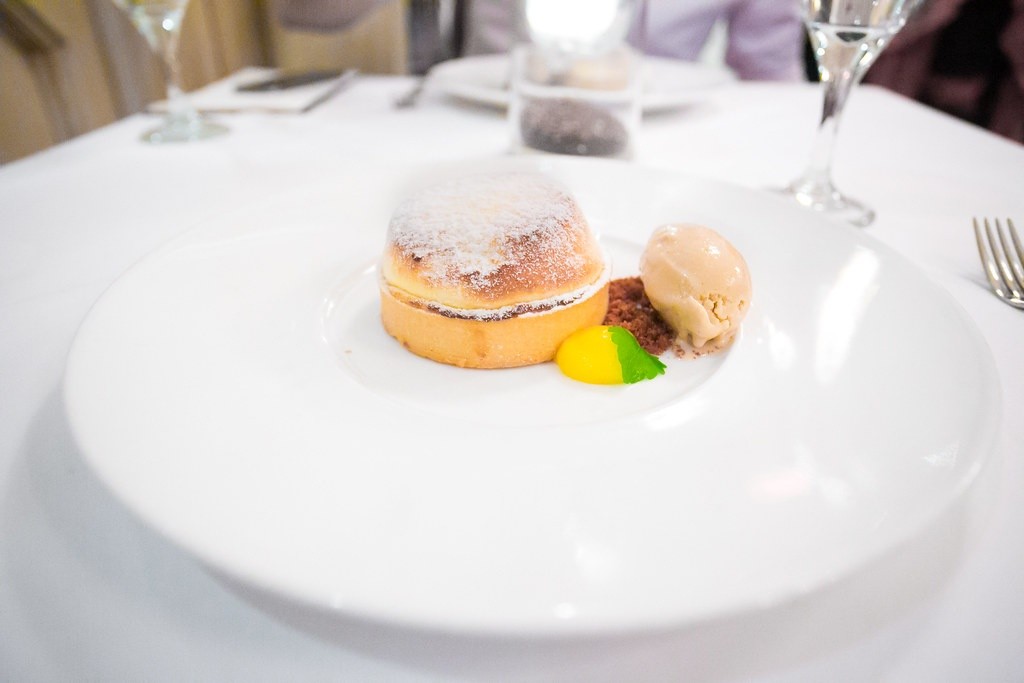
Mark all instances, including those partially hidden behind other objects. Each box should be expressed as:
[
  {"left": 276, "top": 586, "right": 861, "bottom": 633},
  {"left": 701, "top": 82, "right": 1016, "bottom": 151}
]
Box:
[
  {"left": 72, "top": 153, "right": 1010, "bottom": 639},
  {"left": 429, "top": 52, "right": 736, "bottom": 120}
]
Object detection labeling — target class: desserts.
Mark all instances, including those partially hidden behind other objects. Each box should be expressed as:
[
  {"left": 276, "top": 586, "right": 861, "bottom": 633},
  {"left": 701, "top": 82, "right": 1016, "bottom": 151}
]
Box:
[{"left": 381, "top": 171, "right": 610, "bottom": 371}]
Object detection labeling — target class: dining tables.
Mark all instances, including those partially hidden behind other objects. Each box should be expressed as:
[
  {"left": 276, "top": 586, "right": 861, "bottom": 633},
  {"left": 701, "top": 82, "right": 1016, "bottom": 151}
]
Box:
[{"left": 0, "top": 77, "right": 1024, "bottom": 683}]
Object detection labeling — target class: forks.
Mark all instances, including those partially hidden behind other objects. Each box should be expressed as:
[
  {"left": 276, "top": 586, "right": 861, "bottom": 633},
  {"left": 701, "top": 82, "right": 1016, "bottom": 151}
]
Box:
[{"left": 975, "top": 217, "right": 1024, "bottom": 314}]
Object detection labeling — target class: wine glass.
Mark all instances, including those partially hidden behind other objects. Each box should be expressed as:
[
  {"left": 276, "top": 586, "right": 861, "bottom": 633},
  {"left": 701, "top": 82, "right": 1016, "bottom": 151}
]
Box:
[
  {"left": 112, "top": 1, "right": 232, "bottom": 144},
  {"left": 760, "top": 0, "right": 921, "bottom": 223}
]
